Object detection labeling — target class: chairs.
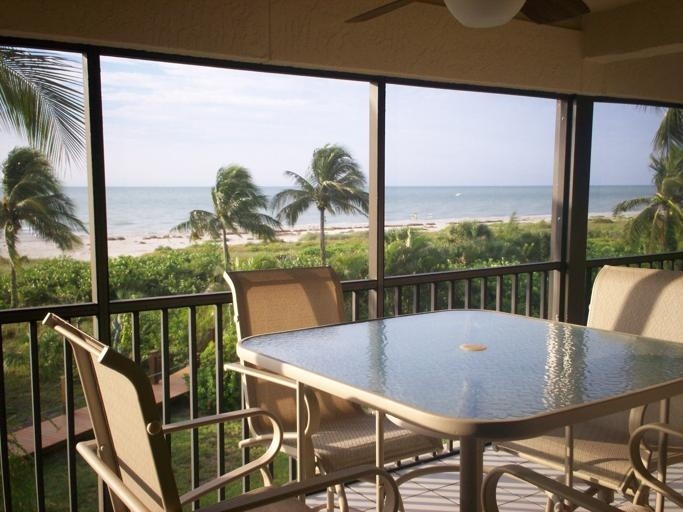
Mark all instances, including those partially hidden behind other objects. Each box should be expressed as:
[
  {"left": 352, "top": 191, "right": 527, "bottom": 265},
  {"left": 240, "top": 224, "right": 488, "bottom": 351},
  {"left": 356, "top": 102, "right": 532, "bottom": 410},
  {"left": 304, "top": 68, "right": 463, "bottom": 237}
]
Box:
[
  {"left": 220, "top": 268, "right": 446, "bottom": 512},
  {"left": 477, "top": 417, "right": 682, "bottom": 512},
  {"left": 42, "top": 314, "right": 404, "bottom": 512},
  {"left": 484, "top": 262, "right": 682, "bottom": 512}
]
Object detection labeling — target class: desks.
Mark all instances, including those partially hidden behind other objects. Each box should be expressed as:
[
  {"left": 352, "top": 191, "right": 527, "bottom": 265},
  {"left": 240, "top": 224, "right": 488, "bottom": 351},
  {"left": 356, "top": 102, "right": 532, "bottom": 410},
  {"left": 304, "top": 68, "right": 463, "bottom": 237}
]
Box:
[{"left": 238, "top": 306, "right": 682, "bottom": 512}]
